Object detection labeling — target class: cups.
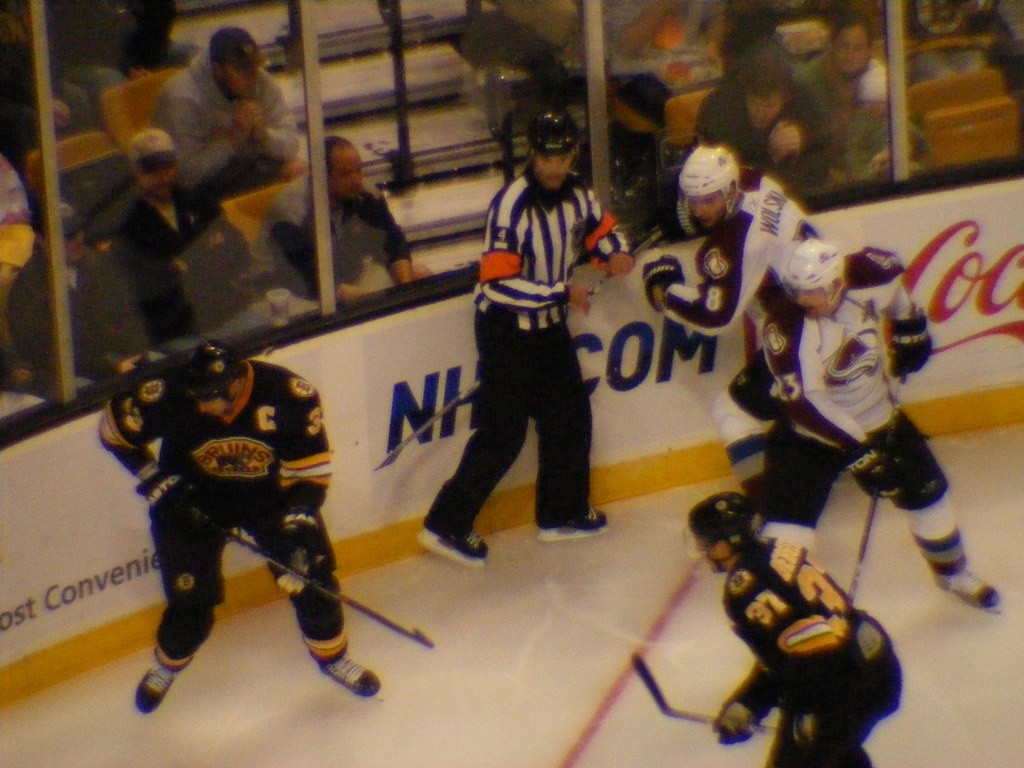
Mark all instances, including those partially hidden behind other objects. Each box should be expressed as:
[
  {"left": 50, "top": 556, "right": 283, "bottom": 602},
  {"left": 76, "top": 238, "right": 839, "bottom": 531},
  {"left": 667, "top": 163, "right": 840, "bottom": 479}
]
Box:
[{"left": 266, "top": 288, "right": 290, "bottom": 327}]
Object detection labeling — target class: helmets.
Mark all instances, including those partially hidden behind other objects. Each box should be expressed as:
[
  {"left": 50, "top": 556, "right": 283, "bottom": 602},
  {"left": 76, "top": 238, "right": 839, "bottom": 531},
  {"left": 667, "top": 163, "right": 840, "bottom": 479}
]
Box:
[
  {"left": 167, "top": 340, "right": 243, "bottom": 401},
  {"left": 783, "top": 239, "right": 847, "bottom": 304},
  {"left": 528, "top": 107, "right": 578, "bottom": 155},
  {"left": 677, "top": 145, "right": 741, "bottom": 213},
  {"left": 687, "top": 491, "right": 761, "bottom": 554}
]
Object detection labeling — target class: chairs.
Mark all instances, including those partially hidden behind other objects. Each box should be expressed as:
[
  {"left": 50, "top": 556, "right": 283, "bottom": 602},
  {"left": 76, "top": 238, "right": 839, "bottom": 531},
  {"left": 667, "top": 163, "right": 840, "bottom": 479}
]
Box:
[
  {"left": 217, "top": 181, "right": 293, "bottom": 250},
  {"left": 22, "top": 129, "right": 128, "bottom": 234},
  {"left": 664, "top": 74, "right": 721, "bottom": 147},
  {"left": 907, "top": 68, "right": 1019, "bottom": 167},
  {"left": 97, "top": 66, "right": 177, "bottom": 151}
]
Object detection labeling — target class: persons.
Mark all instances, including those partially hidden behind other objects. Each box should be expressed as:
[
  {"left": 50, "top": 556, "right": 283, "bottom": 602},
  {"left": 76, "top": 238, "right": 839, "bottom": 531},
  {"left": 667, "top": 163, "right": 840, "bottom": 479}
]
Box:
[
  {"left": 638, "top": 142, "right": 823, "bottom": 525},
  {"left": 416, "top": 104, "right": 636, "bottom": 568},
  {"left": 0, "top": 0, "right": 434, "bottom": 403},
  {"left": 542, "top": 0, "right": 1023, "bottom": 245},
  {"left": 98, "top": 339, "right": 382, "bottom": 713},
  {"left": 687, "top": 491, "right": 902, "bottom": 768},
  {"left": 728, "top": 238, "right": 1001, "bottom": 610}
]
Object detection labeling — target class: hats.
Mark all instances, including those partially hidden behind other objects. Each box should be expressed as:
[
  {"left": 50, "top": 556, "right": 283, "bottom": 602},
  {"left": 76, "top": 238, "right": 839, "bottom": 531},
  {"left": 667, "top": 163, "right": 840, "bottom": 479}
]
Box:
[
  {"left": 207, "top": 26, "right": 266, "bottom": 67},
  {"left": 127, "top": 129, "right": 178, "bottom": 174},
  {"left": 614, "top": 70, "right": 672, "bottom": 127}
]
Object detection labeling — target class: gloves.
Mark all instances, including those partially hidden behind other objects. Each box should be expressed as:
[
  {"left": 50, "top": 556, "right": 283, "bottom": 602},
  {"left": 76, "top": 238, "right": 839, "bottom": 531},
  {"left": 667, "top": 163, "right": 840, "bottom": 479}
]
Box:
[
  {"left": 640, "top": 253, "right": 685, "bottom": 311},
  {"left": 840, "top": 442, "right": 904, "bottom": 500},
  {"left": 267, "top": 506, "right": 334, "bottom": 595},
  {"left": 708, "top": 671, "right": 777, "bottom": 746},
  {"left": 134, "top": 471, "right": 220, "bottom": 543},
  {"left": 889, "top": 311, "right": 935, "bottom": 378}
]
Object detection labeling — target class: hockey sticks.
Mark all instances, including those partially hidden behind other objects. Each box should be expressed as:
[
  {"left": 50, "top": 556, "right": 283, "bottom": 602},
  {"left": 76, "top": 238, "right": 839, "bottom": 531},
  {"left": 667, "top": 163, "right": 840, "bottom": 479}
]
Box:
[
  {"left": 847, "top": 373, "right": 913, "bottom": 605},
  {"left": 373, "top": 228, "right": 664, "bottom": 471},
  {"left": 630, "top": 649, "right": 779, "bottom": 737},
  {"left": 187, "top": 505, "right": 433, "bottom": 651}
]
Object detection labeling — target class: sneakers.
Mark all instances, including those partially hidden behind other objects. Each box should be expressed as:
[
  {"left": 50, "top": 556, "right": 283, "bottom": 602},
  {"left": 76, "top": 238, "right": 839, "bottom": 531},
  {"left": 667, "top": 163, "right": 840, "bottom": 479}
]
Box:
[
  {"left": 417, "top": 526, "right": 489, "bottom": 569},
  {"left": 536, "top": 506, "right": 609, "bottom": 543},
  {"left": 134, "top": 648, "right": 196, "bottom": 716},
  {"left": 932, "top": 569, "right": 1002, "bottom": 616},
  {"left": 312, "top": 646, "right": 382, "bottom": 698}
]
